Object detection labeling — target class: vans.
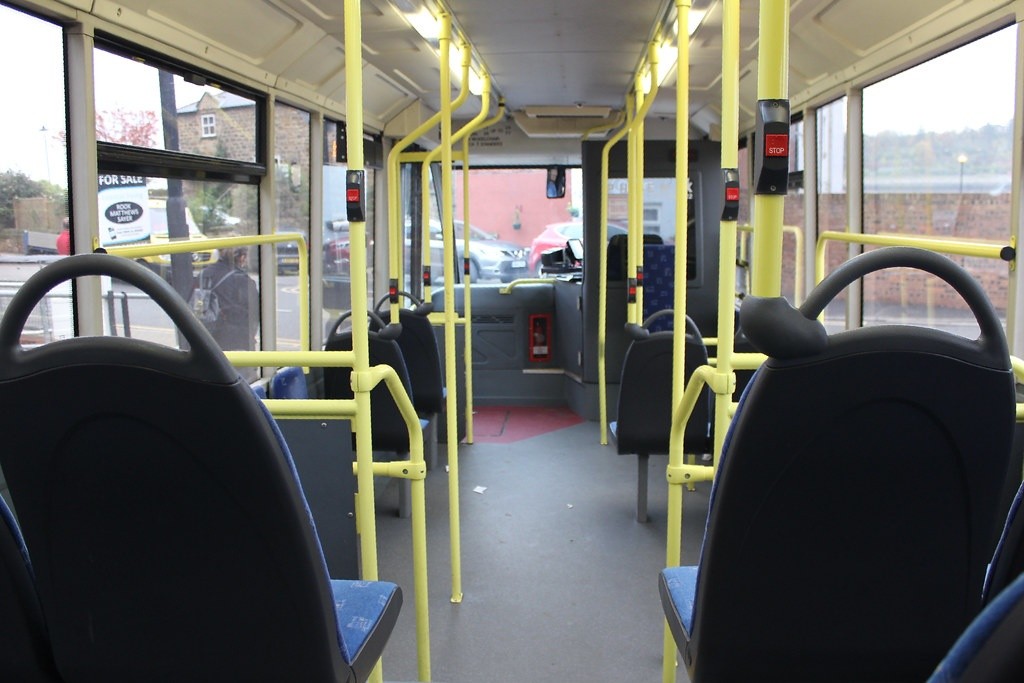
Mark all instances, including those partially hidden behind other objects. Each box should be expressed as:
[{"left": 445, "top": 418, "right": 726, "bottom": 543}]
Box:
[{"left": 93, "top": 198, "right": 219, "bottom": 286}]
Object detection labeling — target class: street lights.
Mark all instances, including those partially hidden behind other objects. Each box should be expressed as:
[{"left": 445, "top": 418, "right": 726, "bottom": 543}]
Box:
[{"left": 957, "top": 154, "right": 968, "bottom": 193}]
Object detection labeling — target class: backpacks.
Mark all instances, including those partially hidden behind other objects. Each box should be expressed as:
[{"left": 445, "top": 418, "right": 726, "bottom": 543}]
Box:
[{"left": 187, "top": 268, "right": 238, "bottom": 334}]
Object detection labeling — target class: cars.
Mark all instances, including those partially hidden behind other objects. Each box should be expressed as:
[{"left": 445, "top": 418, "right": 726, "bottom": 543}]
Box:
[
  {"left": 275, "top": 226, "right": 308, "bottom": 276},
  {"left": 404, "top": 219, "right": 528, "bottom": 284},
  {"left": 528, "top": 220, "right": 628, "bottom": 279},
  {"left": 320, "top": 236, "right": 354, "bottom": 312}
]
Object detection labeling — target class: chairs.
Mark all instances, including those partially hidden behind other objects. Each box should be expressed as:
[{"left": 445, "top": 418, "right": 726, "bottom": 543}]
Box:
[
  {"left": 583, "top": 234, "right": 1024, "bottom": 683},
  {"left": 0, "top": 252, "right": 448, "bottom": 683}
]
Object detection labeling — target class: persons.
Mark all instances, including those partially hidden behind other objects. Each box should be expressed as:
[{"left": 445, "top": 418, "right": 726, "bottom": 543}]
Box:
[
  {"left": 56, "top": 217, "right": 70, "bottom": 256},
  {"left": 197, "top": 245, "right": 259, "bottom": 352},
  {"left": 548, "top": 168, "right": 558, "bottom": 197}
]
